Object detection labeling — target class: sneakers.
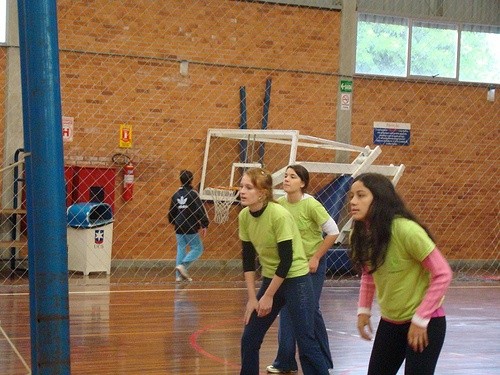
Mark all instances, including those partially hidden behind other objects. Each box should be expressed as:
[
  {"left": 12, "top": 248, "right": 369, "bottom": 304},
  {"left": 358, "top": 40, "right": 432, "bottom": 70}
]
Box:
[{"left": 266, "top": 365, "right": 298, "bottom": 374}]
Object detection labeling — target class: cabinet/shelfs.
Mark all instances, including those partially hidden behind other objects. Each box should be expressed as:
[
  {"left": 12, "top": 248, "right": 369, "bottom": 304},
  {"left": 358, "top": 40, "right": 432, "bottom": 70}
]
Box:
[{"left": 0, "top": 209, "right": 28, "bottom": 248}]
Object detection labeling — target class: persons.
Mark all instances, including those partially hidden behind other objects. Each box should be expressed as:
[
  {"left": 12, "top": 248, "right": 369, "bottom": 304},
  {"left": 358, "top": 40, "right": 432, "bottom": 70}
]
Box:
[
  {"left": 266, "top": 163, "right": 333, "bottom": 373},
  {"left": 237, "top": 166, "right": 329, "bottom": 375},
  {"left": 350, "top": 172, "right": 453, "bottom": 375},
  {"left": 169, "top": 169, "right": 210, "bottom": 282}
]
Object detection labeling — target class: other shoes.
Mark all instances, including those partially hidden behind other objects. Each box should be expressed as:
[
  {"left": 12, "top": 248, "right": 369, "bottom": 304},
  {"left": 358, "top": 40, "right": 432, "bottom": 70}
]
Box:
[
  {"left": 176, "top": 265, "right": 189, "bottom": 279},
  {"left": 176, "top": 277, "right": 192, "bottom": 282}
]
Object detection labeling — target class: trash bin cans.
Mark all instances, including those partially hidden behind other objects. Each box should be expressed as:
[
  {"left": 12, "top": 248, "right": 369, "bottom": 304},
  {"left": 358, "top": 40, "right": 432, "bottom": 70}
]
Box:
[{"left": 67, "top": 203, "right": 115, "bottom": 275}]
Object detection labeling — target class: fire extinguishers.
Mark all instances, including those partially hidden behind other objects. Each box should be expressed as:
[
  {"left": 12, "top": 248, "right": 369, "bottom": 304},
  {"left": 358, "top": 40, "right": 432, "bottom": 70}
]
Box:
[{"left": 111, "top": 153, "right": 135, "bottom": 201}]
